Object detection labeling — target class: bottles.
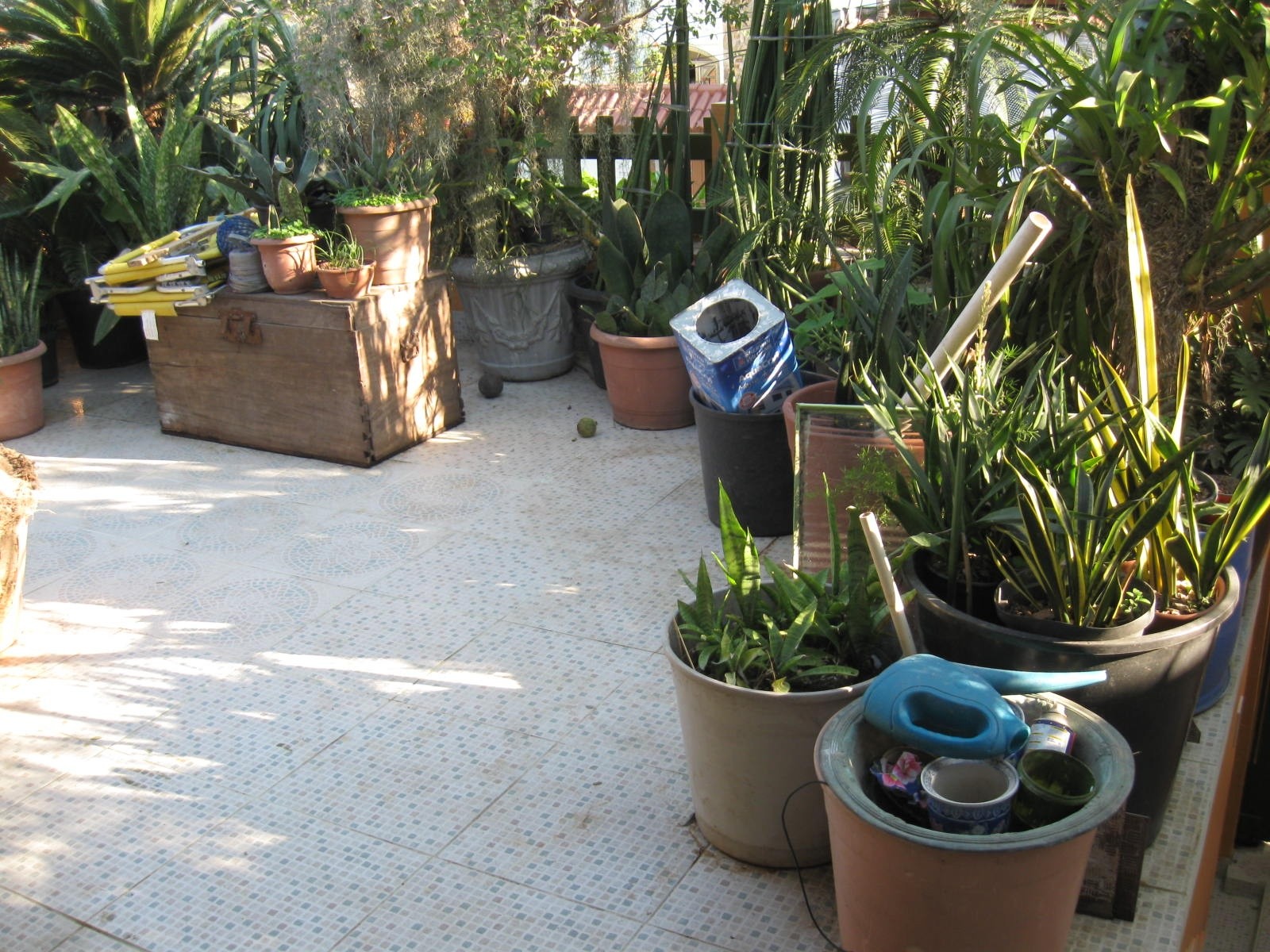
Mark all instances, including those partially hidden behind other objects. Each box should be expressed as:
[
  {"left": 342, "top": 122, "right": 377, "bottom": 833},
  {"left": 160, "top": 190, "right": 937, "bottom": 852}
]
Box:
[{"left": 1024, "top": 702, "right": 1075, "bottom": 758}]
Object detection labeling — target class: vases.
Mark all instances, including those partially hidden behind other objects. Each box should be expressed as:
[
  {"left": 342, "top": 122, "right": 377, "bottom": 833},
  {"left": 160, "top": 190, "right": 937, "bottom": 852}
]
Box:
[
  {"left": 688, "top": 371, "right": 833, "bottom": 536},
  {"left": 811, "top": 690, "right": 1136, "bottom": 951}
]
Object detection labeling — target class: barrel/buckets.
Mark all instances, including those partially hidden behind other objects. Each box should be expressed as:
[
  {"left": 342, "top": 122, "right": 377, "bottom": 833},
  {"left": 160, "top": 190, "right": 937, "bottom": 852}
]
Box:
[
  {"left": 669, "top": 277, "right": 804, "bottom": 415},
  {"left": 689, "top": 372, "right": 835, "bottom": 538}
]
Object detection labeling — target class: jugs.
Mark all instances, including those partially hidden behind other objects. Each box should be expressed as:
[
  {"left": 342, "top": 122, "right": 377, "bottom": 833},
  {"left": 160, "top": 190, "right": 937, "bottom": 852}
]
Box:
[{"left": 859, "top": 654, "right": 1107, "bottom": 760}]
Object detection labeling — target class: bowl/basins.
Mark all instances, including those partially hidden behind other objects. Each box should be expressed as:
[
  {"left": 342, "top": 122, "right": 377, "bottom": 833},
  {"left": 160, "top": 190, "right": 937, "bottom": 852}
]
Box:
[
  {"left": 921, "top": 753, "right": 1019, "bottom": 836},
  {"left": 1012, "top": 748, "right": 1097, "bottom": 829}
]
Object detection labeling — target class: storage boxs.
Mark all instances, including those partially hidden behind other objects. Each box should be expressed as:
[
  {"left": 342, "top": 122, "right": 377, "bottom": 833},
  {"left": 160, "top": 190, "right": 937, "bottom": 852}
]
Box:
[{"left": 146, "top": 272, "right": 465, "bottom": 467}]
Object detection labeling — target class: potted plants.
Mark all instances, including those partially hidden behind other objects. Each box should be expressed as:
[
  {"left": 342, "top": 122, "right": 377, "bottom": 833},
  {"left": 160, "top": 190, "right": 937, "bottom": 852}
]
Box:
[{"left": 0, "top": 0, "right": 1269, "bottom": 868}]
